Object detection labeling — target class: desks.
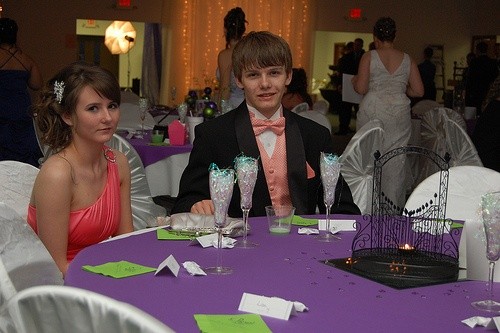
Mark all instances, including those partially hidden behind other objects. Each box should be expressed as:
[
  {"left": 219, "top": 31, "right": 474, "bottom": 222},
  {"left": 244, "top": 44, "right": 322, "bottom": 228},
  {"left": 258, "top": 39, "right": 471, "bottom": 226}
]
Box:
[
  {"left": 63, "top": 213, "right": 500, "bottom": 333},
  {"left": 150, "top": 106, "right": 195, "bottom": 125}
]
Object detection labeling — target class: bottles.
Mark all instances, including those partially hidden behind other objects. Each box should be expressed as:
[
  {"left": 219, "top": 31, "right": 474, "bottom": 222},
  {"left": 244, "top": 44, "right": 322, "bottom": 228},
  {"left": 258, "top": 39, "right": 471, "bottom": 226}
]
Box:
[{"left": 454, "top": 95, "right": 464, "bottom": 115}]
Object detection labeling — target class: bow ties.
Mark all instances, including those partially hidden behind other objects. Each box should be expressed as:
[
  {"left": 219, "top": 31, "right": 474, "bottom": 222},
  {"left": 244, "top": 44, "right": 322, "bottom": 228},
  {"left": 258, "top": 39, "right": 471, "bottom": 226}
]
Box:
[{"left": 250, "top": 117, "right": 285, "bottom": 136}]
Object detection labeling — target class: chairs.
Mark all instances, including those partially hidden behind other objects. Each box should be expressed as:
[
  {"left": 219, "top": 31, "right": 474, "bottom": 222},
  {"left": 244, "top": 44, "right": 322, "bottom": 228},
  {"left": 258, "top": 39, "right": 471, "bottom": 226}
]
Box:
[
  {"left": 0, "top": 135, "right": 175, "bottom": 333},
  {"left": 336, "top": 100, "right": 500, "bottom": 282},
  {"left": 118, "top": 93, "right": 160, "bottom": 127},
  {"left": 297, "top": 101, "right": 332, "bottom": 131}
]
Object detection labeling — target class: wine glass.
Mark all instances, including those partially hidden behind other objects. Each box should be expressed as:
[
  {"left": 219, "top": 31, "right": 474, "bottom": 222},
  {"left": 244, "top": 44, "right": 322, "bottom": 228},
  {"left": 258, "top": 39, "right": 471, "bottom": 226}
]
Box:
[
  {"left": 170, "top": 87, "right": 176, "bottom": 109},
  {"left": 314, "top": 152, "right": 343, "bottom": 242},
  {"left": 137, "top": 97, "right": 149, "bottom": 136},
  {"left": 233, "top": 156, "right": 260, "bottom": 248},
  {"left": 469, "top": 190, "right": 500, "bottom": 313},
  {"left": 203, "top": 168, "right": 235, "bottom": 275}
]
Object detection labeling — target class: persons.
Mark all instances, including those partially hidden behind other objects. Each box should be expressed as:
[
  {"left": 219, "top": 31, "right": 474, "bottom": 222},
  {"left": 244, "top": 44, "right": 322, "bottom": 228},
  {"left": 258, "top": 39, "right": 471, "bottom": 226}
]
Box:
[
  {"left": 351, "top": 16, "right": 423, "bottom": 215},
  {"left": 26, "top": 61, "right": 134, "bottom": 279},
  {"left": 217, "top": 7, "right": 249, "bottom": 114},
  {"left": 170, "top": 31, "right": 361, "bottom": 218},
  {"left": 281, "top": 68, "right": 313, "bottom": 110},
  {"left": 0, "top": 18, "right": 44, "bottom": 169},
  {"left": 463, "top": 42, "right": 500, "bottom": 173},
  {"left": 334, "top": 38, "right": 376, "bottom": 131},
  {"left": 407, "top": 47, "right": 436, "bottom": 108}
]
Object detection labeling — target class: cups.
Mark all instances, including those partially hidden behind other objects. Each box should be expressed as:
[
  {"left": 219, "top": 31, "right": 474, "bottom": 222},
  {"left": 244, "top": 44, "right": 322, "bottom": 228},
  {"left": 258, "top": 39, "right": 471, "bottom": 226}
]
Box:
[
  {"left": 176, "top": 103, "right": 188, "bottom": 123},
  {"left": 150, "top": 130, "right": 164, "bottom": 144},
  {"left": 265, "top": 206, "right": 296, "bottom": 235},
  {"left": 187, "top": 117, "right": 204, "bottom": 144},
  {"left": 464, "top": 107, "right": 476, "bottom": 119}
]
